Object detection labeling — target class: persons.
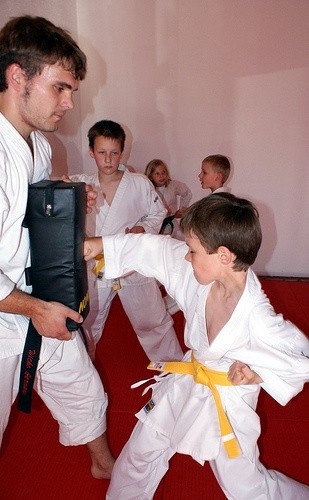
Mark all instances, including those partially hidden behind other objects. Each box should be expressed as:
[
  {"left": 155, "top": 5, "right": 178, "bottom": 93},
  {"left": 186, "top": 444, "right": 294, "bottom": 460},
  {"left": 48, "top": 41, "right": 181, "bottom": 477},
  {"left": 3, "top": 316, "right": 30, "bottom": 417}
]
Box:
[
  {"left": 84, "top": 191, "right": 309, "bottom": 500},
  {"left": 0, "top": 15, "right": 114, "bottom": 479},
  {"left": 178, "top": 154, "right": 230, "bottom": 217},
  {"left": 144, "top": 159, "right": 192, "bottom": 239},
  {"left": 68, "top": 119, "right": 184, "bottom": 363}
]
